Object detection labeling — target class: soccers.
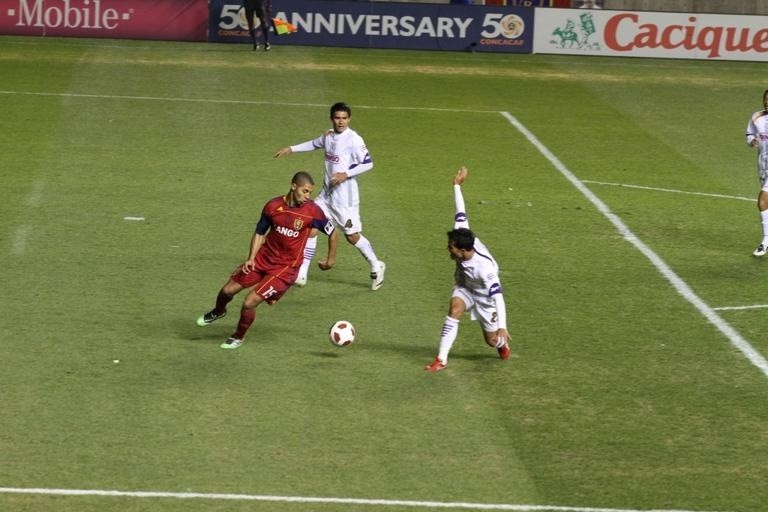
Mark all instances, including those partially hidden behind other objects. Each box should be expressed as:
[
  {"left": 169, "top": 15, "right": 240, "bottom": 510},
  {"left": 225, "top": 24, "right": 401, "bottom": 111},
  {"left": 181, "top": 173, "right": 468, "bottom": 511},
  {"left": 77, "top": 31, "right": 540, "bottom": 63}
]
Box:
[{"left": 328, "top": 319, "right": 356, "bottom": 347}]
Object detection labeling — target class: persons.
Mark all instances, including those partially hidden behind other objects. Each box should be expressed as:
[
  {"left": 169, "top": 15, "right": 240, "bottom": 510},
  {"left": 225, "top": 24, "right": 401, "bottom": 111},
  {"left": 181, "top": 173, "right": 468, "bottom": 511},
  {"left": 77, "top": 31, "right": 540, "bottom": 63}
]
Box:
[
  {"left": 197, "top": 172, "right": 337, "bottom": 349},
  {"left": 746, "top": 90, "right": 768, "bottom": 257},
  {"left": 423, "top": 166, "right": 513, "bottom": 373},
  {"left": 274, "top": 103, "right": 385, "bottom": 290},
  {"left": 243, "top": 0, "right": 272, "bottom": 52}
]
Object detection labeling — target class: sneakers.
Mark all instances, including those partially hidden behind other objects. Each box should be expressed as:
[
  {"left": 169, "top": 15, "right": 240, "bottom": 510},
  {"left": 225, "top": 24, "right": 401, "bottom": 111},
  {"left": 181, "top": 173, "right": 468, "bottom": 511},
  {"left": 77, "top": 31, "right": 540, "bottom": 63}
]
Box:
[
  {"left": 294, "top": 275, "right": 307, "bottom": 286},
  {"left": 265, "top": 41, "right": 271, "bottom": 50},
  {"left": 753, "top": 243, "right": 768, "bottom": 257},
  {"left": 220, "top": 337, "right": 245, "bottom": 349},
  {"left": 424, "top": 356, "right": 446, "bottom": 371},
  {"left": 370, "top": 261, "right": 386, "bottom": 291},
  {"left": 253, "top": 45, "right": 259, "bottom": 50},
  {"left": 498, "top": 343, "right": 511, "bottom": 360},
  {"left": 196, "top": 308, "right": 227, "bottom": 327}
]
